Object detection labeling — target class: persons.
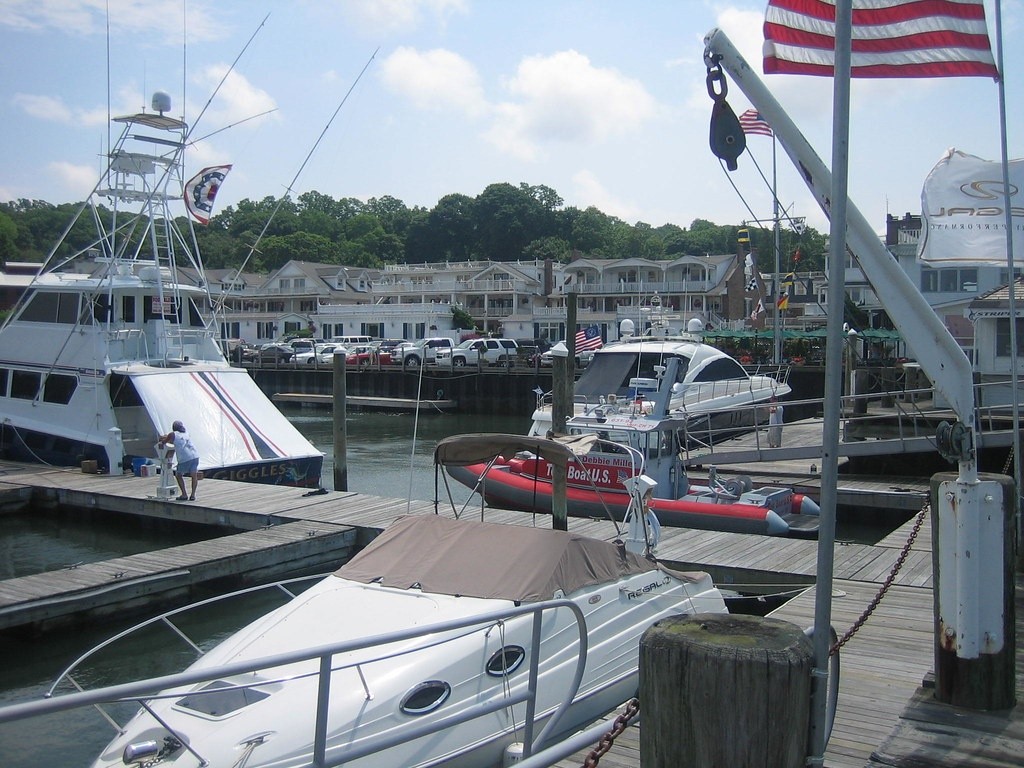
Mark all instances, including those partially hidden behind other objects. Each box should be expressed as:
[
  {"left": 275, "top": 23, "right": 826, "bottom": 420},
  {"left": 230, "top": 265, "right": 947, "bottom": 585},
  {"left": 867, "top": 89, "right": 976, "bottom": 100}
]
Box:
[{"left": 162, "top": 420, "right": 200, "bottom": 500}]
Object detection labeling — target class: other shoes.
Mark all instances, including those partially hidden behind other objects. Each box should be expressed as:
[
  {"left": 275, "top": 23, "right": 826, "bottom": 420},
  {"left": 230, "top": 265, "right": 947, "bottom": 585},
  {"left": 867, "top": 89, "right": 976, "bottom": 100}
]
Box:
[
  {"left": 176, "top": 495, "right": 188, "bottom": 500},
  {"left": 191, "top": 497, "right": 195, "bottom": 500}
]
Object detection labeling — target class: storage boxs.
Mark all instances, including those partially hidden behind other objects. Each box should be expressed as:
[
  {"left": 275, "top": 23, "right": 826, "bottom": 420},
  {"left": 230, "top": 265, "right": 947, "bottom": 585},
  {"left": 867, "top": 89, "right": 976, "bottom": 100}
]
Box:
[
  {"left": 141, "top": 464, "right": 156, "bottom": 477},
  {"left": 81, "top": 460, "right": 97, "bottom": 473}
]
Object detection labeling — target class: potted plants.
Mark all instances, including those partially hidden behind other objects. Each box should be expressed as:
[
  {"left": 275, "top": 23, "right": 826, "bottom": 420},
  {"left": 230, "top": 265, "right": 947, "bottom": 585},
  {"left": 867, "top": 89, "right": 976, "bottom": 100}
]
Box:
[
  {"left": 514, "top": 346, "right": 531, "bottom": 367},
  {"left": 479, "top": 344, "right": 489, "bottom": 367}
]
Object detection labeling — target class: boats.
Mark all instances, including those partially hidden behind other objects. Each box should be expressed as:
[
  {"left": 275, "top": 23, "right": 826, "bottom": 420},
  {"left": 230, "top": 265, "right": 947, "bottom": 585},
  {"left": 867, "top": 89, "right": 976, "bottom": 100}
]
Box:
[
  {"left": 0, "top": 0, "right": 383, "bottom": 487},
  {"left": 44, "top": 430, "right": 728, "bottom": 768},
  {"left": 447, "top": 356, "right": 821, "bottom": 535},
  {"left": 529, "top": 286, "right": 792, "bottom": 457}
]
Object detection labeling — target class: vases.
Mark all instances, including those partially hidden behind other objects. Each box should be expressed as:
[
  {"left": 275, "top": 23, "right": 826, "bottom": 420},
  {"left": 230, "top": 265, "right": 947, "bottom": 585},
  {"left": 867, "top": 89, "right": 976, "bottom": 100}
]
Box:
[
  {"left": 795, "top": 362, "right": 804, "bottom": 366},
  {"left": 742, "top": 361, "right": 752, "bottom": 365}
]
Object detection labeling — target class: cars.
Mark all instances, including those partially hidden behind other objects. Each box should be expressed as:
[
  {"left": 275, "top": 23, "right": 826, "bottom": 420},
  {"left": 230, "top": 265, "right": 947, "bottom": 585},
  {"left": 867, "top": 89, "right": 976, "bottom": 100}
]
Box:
[
  {"left": 366, "top": 340, "right": 386, "bottom": 351},
  {"left": 322, "top": 345, "right": 375, "bottom": 365},
  {"left": 239, "top": 343, "right": 263, "bottom": 360},
  {"left": 248, "top": 345, "right": 294, "bottom": 363},
  {"left": 541, "top": 342, "right": 598, "bottom": 369},
  {"left": 345, "top": 345, "right": 395, "bottom": 364},
  {"left": 396, "top": 343, "right": 415, "bottom": 349}
]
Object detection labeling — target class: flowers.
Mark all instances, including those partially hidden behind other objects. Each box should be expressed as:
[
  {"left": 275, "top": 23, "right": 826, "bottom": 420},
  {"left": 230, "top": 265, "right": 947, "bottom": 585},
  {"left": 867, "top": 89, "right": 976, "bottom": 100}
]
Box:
[
  {"left": 794, "top": 356, "right": 804, "bottom": 362},
  {"left": 740, "top": 356, "right": 753, "bottom": 362}
]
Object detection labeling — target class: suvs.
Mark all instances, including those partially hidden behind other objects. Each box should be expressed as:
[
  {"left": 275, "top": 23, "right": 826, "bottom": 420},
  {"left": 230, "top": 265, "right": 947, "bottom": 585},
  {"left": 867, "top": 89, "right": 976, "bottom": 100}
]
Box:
[
  {"left": 289, "top": 338, "right": 325, "bottom": 354},
  {"left": 517, "top": 337, "right": 553, "bottom": 363},
  {"left": 435, "top": 338, "right": 519, "bottom": 367},
  {"left": 288, "top": 345, "right": 348, "bottom": 365},
  {"left": 389, "top": 337, "right": 455, "bottom": 367},
  {"left": 379, "top": 339, "right": 411, "bottom": 354}
]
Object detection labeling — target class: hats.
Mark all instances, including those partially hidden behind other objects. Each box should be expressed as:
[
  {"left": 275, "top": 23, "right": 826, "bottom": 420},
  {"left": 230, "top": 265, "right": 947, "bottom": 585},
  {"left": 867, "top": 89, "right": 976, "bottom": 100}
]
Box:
[{"left": 173, "top": 420, "right": 185, "bottom": 432}]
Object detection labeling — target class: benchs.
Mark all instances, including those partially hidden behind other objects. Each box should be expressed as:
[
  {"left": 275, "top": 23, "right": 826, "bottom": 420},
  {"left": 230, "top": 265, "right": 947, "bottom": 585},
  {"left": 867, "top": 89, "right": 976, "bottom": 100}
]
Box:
[{"left": 496, "top": 355, "right": 519, "bottom": 367}]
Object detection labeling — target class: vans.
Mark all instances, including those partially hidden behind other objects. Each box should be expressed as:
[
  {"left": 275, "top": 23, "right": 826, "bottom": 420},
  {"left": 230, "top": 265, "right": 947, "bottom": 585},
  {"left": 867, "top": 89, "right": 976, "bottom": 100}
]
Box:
[{"left": 330, "top": 336, "right": 373, "bottom": 346}]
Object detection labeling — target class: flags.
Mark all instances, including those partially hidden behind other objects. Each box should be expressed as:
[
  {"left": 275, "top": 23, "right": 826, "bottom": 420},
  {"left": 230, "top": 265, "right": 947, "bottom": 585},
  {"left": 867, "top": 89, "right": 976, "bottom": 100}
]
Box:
[
  {"left": 915, "top": 146, "right": 1024, "bottom": 268},
  {"left": 574, "top": 325, "right": 602, "bottom": 356},
  {"left": 739, "top": 108, "right": 772, "bottom": 136},
  {"left": 762, "top": 0, "right": 1003, "bottom": 83}
]
